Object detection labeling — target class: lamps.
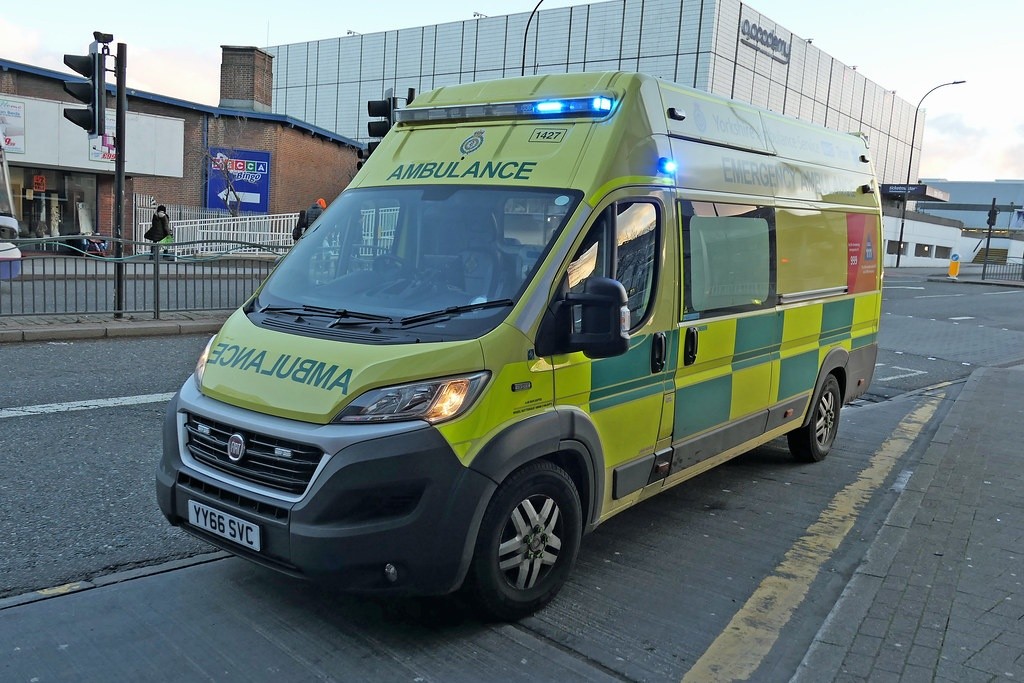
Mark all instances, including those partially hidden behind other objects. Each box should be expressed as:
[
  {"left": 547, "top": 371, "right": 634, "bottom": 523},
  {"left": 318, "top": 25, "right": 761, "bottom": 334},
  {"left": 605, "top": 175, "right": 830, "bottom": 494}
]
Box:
[
  {"left": 804, "top": 38, "right": 814, "bottom": 44},
  {"left": 473, "top": 12, "right": 487, "bottom": 18},
  {"left": 849, "top": 65, "right": 858, "bottom": 70},
  {"left": 347, "top": 30, "right": 361, "bottom": 35}
]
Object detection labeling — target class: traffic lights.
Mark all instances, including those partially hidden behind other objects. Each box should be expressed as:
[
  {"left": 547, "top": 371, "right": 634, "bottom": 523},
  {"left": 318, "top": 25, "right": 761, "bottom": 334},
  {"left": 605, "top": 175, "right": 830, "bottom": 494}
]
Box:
[
  {"left": 986, "top": 208, "right": 998, "bottom": 226},
  {"left": 63, "top": 38, "right": 103, "bottom": 140},
  {"left": 356, "top": 146, "right": 369, "bottom": 170},
  {"left": 367, "top": 86, "right": 395, "bottom": 157}
]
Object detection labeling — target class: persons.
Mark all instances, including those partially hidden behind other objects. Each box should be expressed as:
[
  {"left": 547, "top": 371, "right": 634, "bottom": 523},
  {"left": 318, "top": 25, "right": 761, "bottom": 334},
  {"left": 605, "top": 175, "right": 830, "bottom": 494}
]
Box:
[
  {"left": 149, "top": 205, "right": 175, "bottom": 261},
  {"left": 306, "top": 199, "right": 327, "bottom": 228},
  {"left": 295, "top": 209, "right": 307, "bottom": 242}
]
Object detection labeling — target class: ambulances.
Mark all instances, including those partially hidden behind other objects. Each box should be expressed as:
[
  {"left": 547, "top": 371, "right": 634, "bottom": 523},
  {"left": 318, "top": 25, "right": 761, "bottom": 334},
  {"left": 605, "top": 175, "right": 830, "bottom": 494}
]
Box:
[{"left": 150, "top": 71, "right": 888, "bottom": 623}]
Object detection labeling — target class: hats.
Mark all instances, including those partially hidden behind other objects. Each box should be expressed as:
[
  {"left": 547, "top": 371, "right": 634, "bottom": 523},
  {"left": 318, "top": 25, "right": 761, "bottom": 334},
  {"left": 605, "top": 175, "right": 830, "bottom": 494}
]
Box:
[{"left": 316, "top": 199, "right": 326, "bottom": 209}]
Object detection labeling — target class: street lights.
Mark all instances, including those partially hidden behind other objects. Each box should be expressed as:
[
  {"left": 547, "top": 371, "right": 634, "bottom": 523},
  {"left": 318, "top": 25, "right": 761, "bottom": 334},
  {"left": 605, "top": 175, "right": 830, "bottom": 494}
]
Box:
[{"left": 894, "top": 80, "right": 967, "bottom": 268}]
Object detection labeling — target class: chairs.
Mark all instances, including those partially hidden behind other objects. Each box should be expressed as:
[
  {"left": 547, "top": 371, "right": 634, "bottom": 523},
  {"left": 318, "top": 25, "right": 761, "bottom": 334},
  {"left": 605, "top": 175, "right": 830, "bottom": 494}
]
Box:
[{"left": 441, "top": 205, "right": 502, "bottom": 298}]
